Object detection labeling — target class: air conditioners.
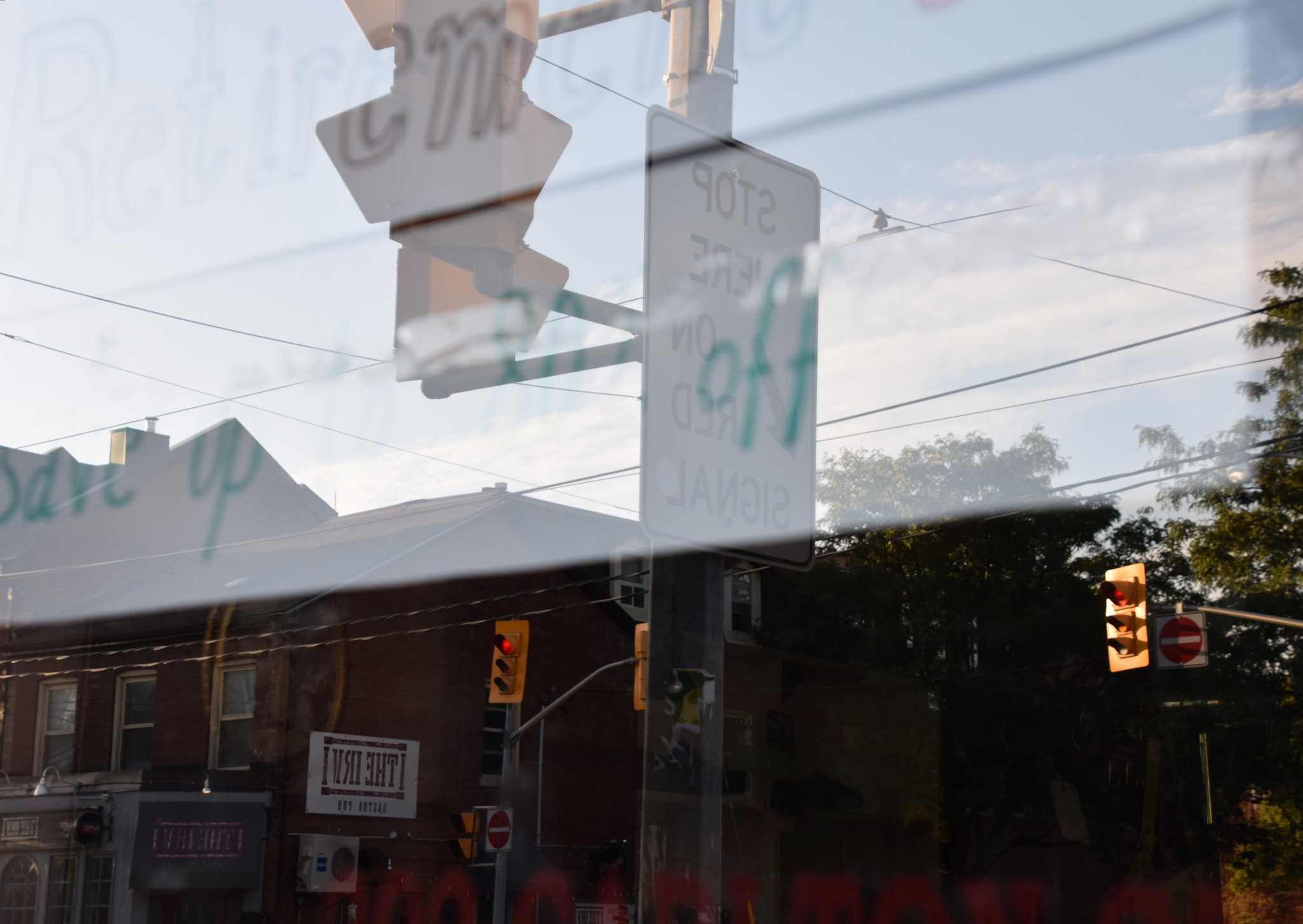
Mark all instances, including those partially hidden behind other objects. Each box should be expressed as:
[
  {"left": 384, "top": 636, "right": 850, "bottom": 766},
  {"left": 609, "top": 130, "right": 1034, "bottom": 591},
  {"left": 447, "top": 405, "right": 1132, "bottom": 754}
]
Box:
[{"left": 298, "top": 835, "right": 360, "bottom": 893}]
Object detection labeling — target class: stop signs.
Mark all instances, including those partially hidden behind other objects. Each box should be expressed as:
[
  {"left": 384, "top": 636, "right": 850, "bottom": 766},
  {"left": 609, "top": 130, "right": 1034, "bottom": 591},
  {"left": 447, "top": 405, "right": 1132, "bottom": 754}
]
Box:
[
  {"left": 1157, "top": 616, "right": 1202, "bottom": 664},
  {"left": 488, "top": 809, "right": 512, "bottom": 849}
]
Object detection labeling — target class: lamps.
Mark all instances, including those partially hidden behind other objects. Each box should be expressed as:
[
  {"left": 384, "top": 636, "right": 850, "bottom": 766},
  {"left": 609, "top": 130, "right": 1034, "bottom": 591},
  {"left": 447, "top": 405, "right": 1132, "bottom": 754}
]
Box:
[
  {"left": 855, "top": 208, "right": 905, "bottom": 241},
  {"left": 202, "top": 775, "right": 211, "bottom": 794},
  {"left": 34, "top": 767, "right": 82, "bottom": 796}
]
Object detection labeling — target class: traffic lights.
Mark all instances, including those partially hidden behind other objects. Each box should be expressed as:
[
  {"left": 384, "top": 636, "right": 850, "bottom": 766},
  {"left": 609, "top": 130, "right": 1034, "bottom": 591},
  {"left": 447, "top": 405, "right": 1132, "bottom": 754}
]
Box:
[
  {"left": 1100, "top": 563, "right": 1149, "bottom": 671},
  {"left": 487, "top": 618, "right": 530, "bottom": 705},
  {"left": 71, "top": 812, "right": 103, "bottom": 845},
  {"left": 444, "top": 810, "right": 483, "bottom": 863}
]
